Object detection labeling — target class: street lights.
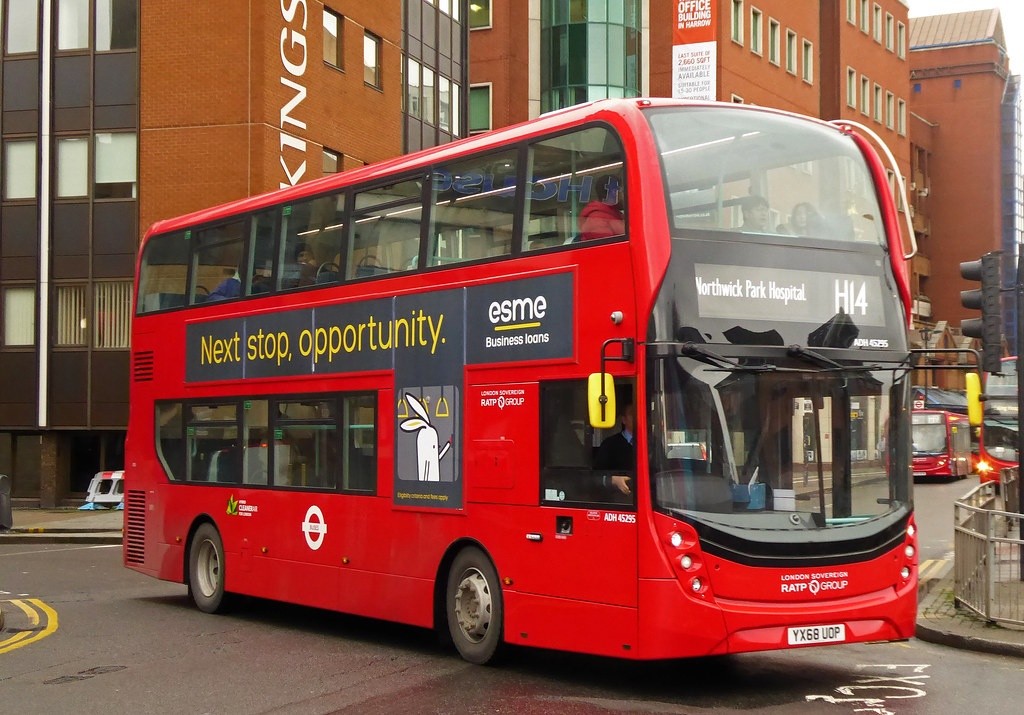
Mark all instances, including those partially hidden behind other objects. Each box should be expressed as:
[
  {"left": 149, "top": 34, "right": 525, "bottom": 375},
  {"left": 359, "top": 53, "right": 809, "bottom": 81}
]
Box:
[{"left": 920, "top": 326, "right": 944, "bottom": 409}]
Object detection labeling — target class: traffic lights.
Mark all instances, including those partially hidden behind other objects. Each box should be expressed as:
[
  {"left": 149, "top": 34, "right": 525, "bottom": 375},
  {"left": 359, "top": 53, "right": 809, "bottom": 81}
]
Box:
[{"left": 959, "top": 252, "right": 1003, "bottom": 375}]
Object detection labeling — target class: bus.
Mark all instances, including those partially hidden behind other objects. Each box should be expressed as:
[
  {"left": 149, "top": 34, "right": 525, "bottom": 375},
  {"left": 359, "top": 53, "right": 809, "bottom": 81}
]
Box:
[
  {"left": 880, "top": 410, "right": 982, "bottom": 483},
  {"left": 120, "top": 94, "right": 992, "bottom": 670},
  {"left": 978, "top": 355, "right": 1021, "bottom": 495}
]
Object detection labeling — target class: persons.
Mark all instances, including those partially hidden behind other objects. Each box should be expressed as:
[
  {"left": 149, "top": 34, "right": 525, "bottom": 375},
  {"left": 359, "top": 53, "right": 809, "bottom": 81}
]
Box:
[
  {"left": 298, "top": 238, "right": 338, "bottom": 287},
  {"left": 207, "top": 263, "right": 269, "bottom": 301},
  {"left": 725, "top": 197, "right": 770, "bottom": 233},
  {"left": 580, "top": 175, "right": 625, "bottom": 240},
  {"left": 790, "top": 202, "right": 821, "bottom": 230},
  {"left": 592, "top": 398, "right": 662, "bottom": 496}
]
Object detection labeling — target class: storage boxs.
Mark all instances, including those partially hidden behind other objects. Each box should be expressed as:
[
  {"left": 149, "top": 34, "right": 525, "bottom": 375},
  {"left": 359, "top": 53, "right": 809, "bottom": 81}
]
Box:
[{"left": 773, "top": 489, "right": 796, "bottom": 511}]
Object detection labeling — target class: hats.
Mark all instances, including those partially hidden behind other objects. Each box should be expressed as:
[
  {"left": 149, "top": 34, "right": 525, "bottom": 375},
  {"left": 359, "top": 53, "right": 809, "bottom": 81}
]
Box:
[{"left": 295, "top": 243, "right": 314, "bottom": 262}]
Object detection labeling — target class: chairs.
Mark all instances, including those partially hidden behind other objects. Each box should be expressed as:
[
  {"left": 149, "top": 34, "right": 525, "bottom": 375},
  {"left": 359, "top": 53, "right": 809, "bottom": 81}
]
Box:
[
  {"left": 194, "top": 285, "right": 211, "bottom": 304},
  {"left": 206, "top": 449, "right": 241, "bottom": 481},
  {"left": 284, "top": 235, "right": 581, "bottom": 289},
  {"left": 546, "top": 414, "right": 588, "bottom": 466}
]
